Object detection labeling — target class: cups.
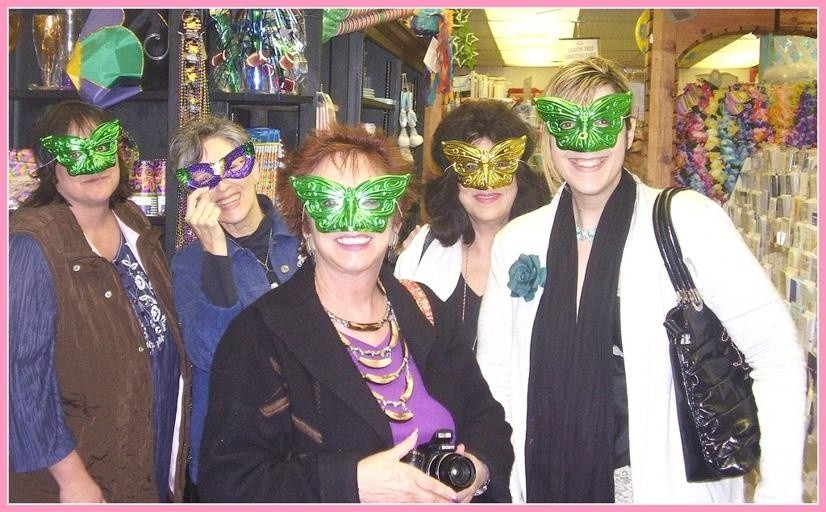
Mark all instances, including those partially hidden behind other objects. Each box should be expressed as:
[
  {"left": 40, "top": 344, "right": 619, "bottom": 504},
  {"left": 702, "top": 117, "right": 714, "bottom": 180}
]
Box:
[{"left": 126, "top": 158, "right": 167, "bottom": 216}]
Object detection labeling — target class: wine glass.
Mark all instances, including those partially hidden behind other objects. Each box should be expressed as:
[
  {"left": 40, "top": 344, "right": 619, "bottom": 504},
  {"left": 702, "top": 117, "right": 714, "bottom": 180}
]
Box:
[{"left": 25, "top": 13, "right": 69, "bottom": 91}]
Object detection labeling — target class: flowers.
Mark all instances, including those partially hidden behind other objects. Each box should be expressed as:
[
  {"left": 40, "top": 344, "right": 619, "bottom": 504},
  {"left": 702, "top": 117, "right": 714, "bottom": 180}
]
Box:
[{"left": 508, "top": 253, "right": 548, "bottom": 302}]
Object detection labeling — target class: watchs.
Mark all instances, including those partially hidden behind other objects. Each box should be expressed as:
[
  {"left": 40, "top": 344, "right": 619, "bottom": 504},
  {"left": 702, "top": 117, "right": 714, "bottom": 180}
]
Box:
[{"left": 474, "top": 469, "right": 491, "bottom": 498}]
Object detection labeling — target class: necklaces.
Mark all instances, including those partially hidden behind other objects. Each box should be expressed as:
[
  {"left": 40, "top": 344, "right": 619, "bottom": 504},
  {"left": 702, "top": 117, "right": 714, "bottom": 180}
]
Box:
[
  {"left": 327, "top": 293, "right": 413, "bottom": 422},
  {"left": 576, "top": 227, "right": 596, "bottom": 240},
  {"left": 463, "top": 242, "right": 477, "bottom": 349},
  {"left": 225, "top": 229, "right": 274, "bottom": 275}
]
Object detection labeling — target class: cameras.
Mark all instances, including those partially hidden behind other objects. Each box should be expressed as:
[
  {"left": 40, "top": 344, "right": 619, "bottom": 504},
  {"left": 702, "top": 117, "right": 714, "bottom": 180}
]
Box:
[{"left": 398, "top": 428, "right": 477, "bottom": 494}]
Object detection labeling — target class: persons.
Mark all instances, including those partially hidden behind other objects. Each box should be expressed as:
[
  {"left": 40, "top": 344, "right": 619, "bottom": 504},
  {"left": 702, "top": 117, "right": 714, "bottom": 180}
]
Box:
[
  {"left": 196, "top": 121, "right": 515, "bottom": 504},
  {"left": 170, "top": 118, "right": 305, "bottom": 502},
  {"left": 477, "top": 59, "right": 808, "bottom": 504},
  {"left": 393, "top": 97, "right": 552, "bottom": 353},
  {"left": 8, "top": 99, "right": 193, "bottom": 503}
]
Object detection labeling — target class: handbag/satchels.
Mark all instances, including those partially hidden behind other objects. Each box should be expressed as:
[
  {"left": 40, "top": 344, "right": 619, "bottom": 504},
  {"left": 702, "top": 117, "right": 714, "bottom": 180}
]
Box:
[{"left": 652, "top": 186, "right": 816, "bottom": 480}]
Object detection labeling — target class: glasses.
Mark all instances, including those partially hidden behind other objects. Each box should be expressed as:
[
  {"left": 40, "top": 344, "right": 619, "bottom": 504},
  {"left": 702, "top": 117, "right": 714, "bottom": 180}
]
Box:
[{"left": 175, "top": 144, "right": 253, "bottom": 189}]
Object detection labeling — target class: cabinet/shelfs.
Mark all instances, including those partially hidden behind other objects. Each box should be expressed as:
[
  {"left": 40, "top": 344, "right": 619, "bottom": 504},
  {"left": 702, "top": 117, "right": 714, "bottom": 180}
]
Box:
[
  {"left": 326, "top": 14, "right": 427, "bottom": 248},
  {"left": 8, "top": 6, "right": 327, "bottom": 263}
]
